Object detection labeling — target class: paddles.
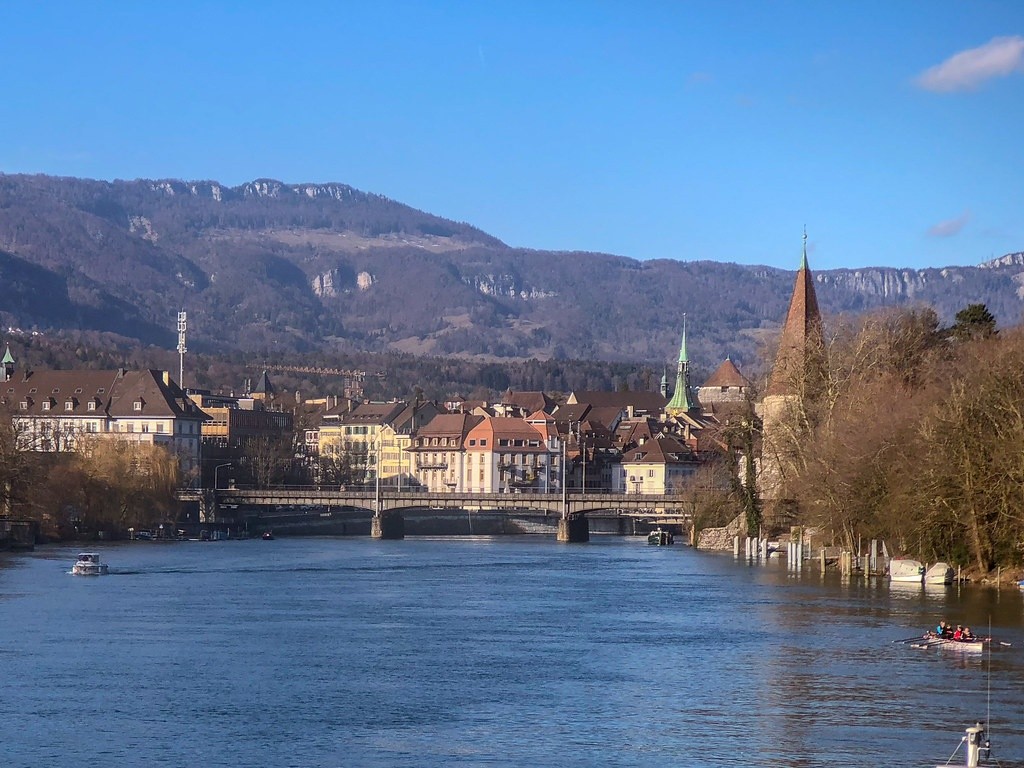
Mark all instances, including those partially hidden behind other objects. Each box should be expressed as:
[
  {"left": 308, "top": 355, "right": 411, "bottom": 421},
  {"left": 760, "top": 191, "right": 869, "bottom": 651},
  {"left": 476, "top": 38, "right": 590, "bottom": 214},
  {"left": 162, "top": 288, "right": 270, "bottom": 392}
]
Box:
[
  {"left": 973, "top": 633, "right": 1012, "bottom": 648},
  {"left": 891, "top": 634, "right": 949, "bottom": 649}
]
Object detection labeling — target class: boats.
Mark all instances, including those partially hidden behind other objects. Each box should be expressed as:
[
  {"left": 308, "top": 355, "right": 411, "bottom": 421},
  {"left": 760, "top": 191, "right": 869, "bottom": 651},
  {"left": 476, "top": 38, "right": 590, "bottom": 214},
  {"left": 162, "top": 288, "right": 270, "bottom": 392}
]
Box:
[
  {"left": 262, "top": 533, "right": 272, "bottom": 540},
  {"left": 926, "top": 630, "right": 1003, "bottom": 654},
  {"left": 72, "top": 553, "right": 108, "bottom": 576},
  {"left": 647, "top": 530, "right": 674, "bottom": 544},
  {"left": 923, "top": 562, "right": 953, "bottom": 585},
  {"left": 888, "top": 559, "right": 924, "bottom": 582}
]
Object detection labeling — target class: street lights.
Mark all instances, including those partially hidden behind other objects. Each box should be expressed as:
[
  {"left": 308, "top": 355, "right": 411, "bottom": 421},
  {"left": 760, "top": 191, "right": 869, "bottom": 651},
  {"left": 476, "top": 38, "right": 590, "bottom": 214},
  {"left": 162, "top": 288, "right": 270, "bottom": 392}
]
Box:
[{"left": 214, "top": 463, "right": 231, "bottom": 490}]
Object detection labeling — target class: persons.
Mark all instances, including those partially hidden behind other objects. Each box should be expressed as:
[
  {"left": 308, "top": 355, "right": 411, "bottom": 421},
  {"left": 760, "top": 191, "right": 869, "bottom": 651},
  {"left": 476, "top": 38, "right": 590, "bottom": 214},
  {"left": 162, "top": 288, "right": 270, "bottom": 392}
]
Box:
[{"left": 937, "top": 620, "right": 973, "bottom": 642}]
